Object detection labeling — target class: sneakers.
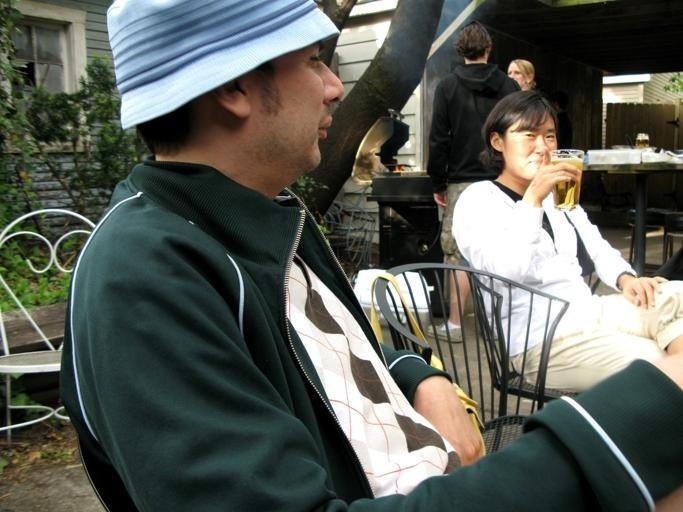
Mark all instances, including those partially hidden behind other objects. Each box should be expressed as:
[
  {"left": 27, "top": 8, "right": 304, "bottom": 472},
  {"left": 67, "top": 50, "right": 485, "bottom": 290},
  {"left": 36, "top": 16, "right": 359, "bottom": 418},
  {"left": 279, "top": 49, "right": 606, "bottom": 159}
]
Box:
[{"left": 427, "top": 324, "right": 464, "bottom": 344}]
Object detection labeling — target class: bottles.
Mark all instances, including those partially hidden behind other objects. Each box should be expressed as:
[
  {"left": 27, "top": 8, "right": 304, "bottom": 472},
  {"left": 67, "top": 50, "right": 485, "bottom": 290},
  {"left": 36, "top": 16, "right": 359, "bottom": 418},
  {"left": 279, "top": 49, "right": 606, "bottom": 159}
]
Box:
[{"left": 635, "top": 133, "right": 650, "bottom": 149}]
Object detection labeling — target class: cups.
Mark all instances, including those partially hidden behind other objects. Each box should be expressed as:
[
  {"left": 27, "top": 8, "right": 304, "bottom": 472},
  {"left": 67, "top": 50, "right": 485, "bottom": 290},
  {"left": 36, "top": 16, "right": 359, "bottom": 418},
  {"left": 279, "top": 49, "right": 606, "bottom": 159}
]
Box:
[{"left": 551, "top": 148, "right": 585, "bottom": 211}]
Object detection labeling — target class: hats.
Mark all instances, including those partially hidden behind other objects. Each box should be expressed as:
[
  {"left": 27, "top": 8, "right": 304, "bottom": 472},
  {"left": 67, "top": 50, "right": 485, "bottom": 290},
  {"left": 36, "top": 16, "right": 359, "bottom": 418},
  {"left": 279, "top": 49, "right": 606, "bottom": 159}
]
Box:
[{"left": 106, "top": 1, "right": 340, "bottom": 131}]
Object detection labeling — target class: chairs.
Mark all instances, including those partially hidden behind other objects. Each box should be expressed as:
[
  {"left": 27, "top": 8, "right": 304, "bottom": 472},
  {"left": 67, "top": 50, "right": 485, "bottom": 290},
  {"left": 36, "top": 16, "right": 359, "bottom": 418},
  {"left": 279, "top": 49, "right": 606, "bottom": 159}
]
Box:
[
  {"left": 0, "top": 205, "right": 100, "bottom": 444},
  {"left": 373, "top": 254, "right": 582, "bottom": 471}
]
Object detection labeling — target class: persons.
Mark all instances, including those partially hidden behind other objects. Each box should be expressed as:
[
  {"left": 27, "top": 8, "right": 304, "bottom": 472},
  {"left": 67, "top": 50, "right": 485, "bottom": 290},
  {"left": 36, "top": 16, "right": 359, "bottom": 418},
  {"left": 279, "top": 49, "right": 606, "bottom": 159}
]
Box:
[
  {"left": 426, "top": 19, "right": 520, "bottom": 344},
  {"left": 59, "top": 0, "right": 682, "bottom": 512},
  {"left": 507, "top": 59, "right": 537, "bottom": 90},
  {"left": 449, "top": 89, "right": 683, "bottom": 395}
]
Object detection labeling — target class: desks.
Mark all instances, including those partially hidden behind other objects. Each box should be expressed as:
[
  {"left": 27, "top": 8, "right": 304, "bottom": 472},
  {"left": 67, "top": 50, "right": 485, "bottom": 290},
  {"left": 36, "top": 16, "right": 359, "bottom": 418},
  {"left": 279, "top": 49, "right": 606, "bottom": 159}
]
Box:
[{"left": 580, "top": 161, "right": 683, "bottom": 273}]
